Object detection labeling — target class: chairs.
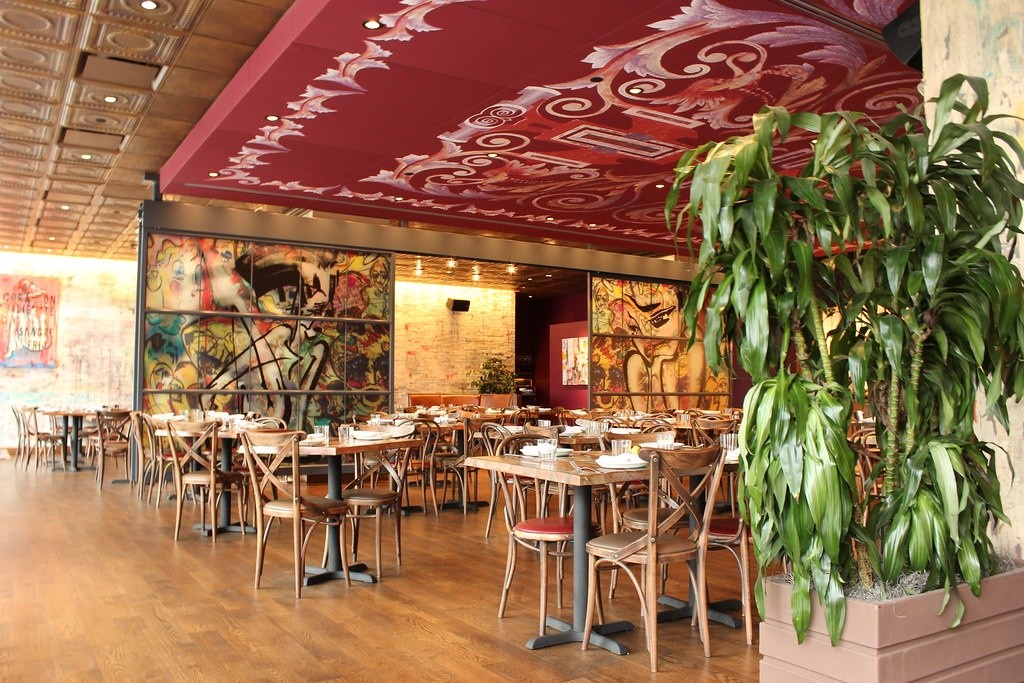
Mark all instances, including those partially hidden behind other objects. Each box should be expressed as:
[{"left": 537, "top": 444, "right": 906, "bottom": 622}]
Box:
[
  {"left": 11, "top": 393, "right": 440, "bottom": 600},
  {"left": 427, "top": 395, "right": 874, "bottom": 675}
]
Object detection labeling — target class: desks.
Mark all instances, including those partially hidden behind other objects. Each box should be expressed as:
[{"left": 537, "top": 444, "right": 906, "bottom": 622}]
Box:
[{"left": 42, "top": 408, "right": 878, "bottom": 656}]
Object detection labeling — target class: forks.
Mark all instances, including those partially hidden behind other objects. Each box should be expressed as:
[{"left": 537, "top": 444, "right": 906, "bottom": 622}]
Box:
[{"left": 569, "top": 461, "right": 605, "bottom": 474}]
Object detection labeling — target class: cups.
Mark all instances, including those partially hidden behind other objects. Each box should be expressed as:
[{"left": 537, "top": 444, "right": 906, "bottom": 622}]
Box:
[
  {"left": 511, "top": 405, "right": 739, "bottom": 459},
  {"left": 313, "top": 404, "right": 467, "bottom": 447},
  {"left": 180, "top": 408, "right": 241, "bottom": 433}
]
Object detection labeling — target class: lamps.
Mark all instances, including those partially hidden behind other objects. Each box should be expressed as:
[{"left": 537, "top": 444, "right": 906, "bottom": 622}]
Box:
[
  {"left": 446, "top": 298, "right": 471, "bottom": 311},
  {"left": 879, "top": 3, "right": 923, "bottom": 74}
]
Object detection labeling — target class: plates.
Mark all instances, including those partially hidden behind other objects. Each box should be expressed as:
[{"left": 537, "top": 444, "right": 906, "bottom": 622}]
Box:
[
  {"left": 610, "top": 427, "right": 640, "bottom": 434},
  {"left": 519, "top": 449, "right": 573, "bottom": 456},
  {"left": 498, "top": 426, "right": 523, "bottom": 435},
  {"left": 352, "top": 431, "right": 391, "bottom": 439},
  {"left": 557, "top": 432, "right": 580, "bottom": 437},
  {"left": 298, "top": 433, "right": 324, "bottom": 445},
  {"left": 593, "top": 458, "right": 649, "bottom": 469}
]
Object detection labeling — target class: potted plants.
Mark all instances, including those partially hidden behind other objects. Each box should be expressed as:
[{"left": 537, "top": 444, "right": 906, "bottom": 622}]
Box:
[{"left": 666, "top": 72, "right": 1024, "bottom": 683}]
